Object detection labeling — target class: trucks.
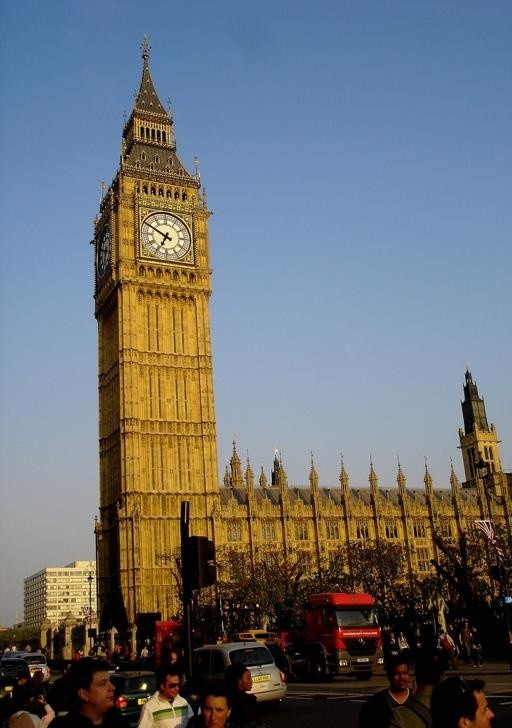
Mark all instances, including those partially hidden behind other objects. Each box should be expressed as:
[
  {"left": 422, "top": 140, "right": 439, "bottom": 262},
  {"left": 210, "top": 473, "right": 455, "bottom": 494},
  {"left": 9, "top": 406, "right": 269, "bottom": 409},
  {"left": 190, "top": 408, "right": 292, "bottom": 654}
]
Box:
[{"left": 234, "top": 592, "right": 385, "bottom": 680}]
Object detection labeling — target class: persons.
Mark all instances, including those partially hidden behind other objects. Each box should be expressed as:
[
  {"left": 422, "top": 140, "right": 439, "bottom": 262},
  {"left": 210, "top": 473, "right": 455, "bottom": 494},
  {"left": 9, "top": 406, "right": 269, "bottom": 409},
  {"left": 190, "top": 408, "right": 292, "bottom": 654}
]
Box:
[
  {"left": 0, "top": 633, "right": 259, "bottom": 728},
  {"left": 429, "top": 676, "right": 495, "bottom": 728},
  {"left": 361, "top": 656, "right": 433, "bottom": 728},
  {"left": 388, "top": 618, "right": 483, "bottom": 670}
]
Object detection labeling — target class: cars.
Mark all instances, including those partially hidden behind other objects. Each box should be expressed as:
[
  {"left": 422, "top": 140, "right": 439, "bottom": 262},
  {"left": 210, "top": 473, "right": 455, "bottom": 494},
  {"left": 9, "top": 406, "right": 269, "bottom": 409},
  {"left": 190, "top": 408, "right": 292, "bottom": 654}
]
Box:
[
  {"left": 63, "top": 657, "right": 120, "bottom": 674},
  {"left": 109, "top": 670, "right": 159, "bottom": 720},
  {"left": 1, "top": 652, "right": 50, "bottom": 693},
  {"left": 174, "top": 643, "right": 289, "bottom": 704}
]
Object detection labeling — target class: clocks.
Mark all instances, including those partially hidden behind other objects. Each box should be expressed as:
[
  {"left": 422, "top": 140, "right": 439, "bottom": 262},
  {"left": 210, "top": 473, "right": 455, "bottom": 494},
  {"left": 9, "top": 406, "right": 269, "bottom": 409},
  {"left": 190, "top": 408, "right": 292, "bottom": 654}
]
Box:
[
  {"left": 95, "top": 224, "right": 112, "bottom": 277},
  {"left": 140, "top": 210, "right": 194, "bottom": 261}
]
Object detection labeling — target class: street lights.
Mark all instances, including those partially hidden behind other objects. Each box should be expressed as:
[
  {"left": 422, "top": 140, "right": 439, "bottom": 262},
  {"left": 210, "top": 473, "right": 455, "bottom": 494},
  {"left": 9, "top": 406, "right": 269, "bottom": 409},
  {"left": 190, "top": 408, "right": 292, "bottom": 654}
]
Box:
[{"left": 86, "top": 572, "right": 95, "bottom": 654}]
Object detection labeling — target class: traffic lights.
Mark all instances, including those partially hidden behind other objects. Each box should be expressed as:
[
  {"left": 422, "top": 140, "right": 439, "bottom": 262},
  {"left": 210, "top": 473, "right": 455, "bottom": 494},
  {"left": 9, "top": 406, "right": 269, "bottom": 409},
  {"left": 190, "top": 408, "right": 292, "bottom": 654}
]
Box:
[{"left": 190, "top": 537, "right": 216, "bottom": 590}]
